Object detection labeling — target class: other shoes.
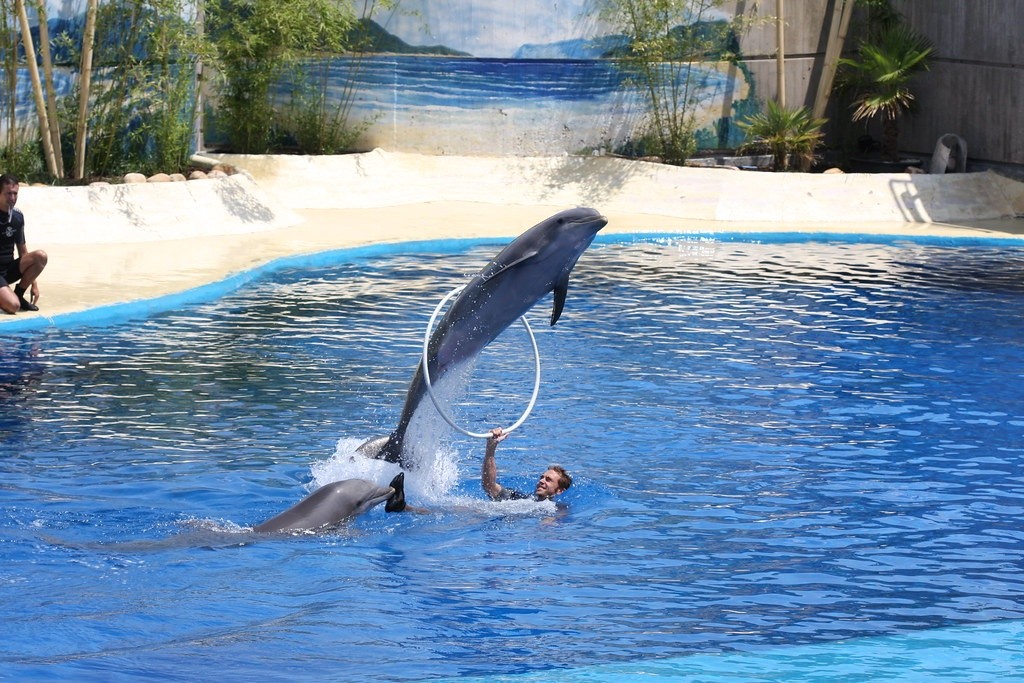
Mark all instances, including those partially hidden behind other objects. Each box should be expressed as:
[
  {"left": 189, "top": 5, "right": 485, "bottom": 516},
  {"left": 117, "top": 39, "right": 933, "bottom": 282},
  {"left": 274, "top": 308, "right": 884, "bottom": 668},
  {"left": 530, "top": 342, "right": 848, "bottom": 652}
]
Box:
[
  {"left": 385, "top": 472, "right": 407, "bottom": 513},
  {"left": 14, "top": 285, "right": 39, "bottom": 311}
]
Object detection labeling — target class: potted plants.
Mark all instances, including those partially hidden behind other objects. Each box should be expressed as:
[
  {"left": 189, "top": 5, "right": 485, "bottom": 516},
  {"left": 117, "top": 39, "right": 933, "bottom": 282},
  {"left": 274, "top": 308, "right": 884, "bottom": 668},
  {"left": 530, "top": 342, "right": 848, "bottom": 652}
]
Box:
[{"left": 830, "top": 19, "right": 943, "bottom": 173}]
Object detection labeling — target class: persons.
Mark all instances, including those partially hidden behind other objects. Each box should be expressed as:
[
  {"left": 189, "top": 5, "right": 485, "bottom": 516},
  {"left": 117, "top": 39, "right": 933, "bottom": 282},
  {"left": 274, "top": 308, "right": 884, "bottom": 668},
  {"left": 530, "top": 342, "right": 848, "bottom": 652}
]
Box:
[
  {"left": 385, "top": 427, "right": 570, "bottom": 532},
  {"left": 1, "top": 174, "right": 48, "bottom": 315}
]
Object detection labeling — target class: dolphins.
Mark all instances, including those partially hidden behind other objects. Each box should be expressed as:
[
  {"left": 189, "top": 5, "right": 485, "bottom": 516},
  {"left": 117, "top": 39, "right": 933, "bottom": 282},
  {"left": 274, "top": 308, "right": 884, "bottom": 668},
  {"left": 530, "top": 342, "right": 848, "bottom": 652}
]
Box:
[
  {"left": 373, "top": 206, "right": 608, "bottom": 466},
  {"left": 40, "top": 477, "right": 396, "bottom": 556}
]
